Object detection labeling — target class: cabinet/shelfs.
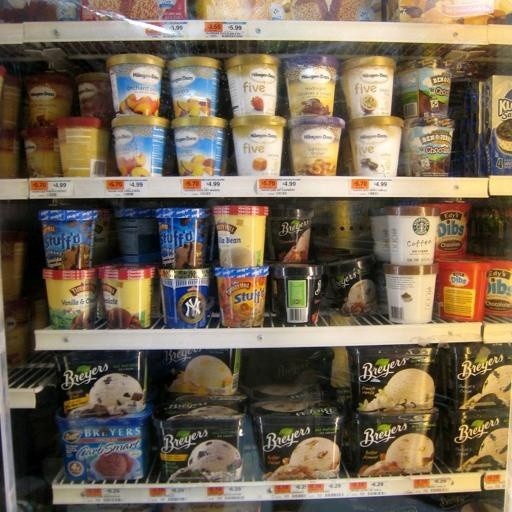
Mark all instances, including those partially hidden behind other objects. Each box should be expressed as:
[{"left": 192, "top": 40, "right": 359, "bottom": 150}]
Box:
[{"left": 0, "top": 0, "right": 511, "bottom": 512}]
